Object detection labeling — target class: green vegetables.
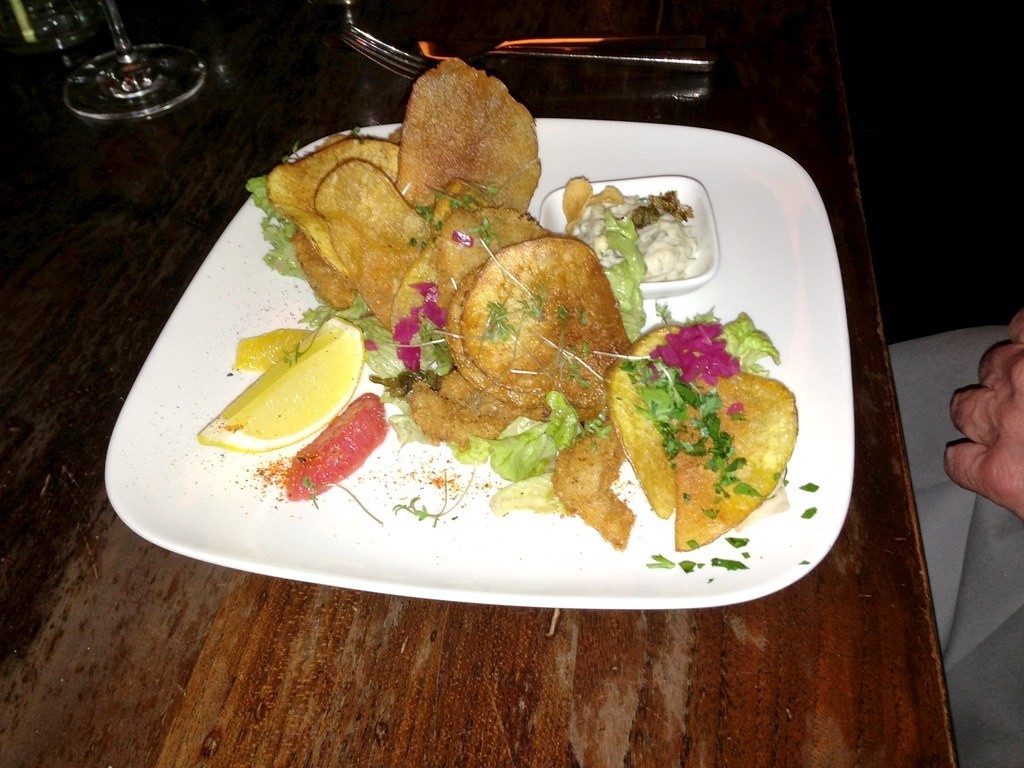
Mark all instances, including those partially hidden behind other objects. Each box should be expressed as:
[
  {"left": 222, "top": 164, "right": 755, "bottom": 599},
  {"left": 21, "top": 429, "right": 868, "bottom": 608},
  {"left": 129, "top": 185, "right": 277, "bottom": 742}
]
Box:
[{"left": 246, "top": 123, "right": 818, "bottom": 575}]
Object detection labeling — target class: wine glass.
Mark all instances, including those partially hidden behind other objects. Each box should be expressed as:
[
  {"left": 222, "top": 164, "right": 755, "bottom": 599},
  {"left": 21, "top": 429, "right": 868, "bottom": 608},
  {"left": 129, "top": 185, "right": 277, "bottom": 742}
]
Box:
[{"left": 62, "top": 0, "right": 207, "bottom": 121}]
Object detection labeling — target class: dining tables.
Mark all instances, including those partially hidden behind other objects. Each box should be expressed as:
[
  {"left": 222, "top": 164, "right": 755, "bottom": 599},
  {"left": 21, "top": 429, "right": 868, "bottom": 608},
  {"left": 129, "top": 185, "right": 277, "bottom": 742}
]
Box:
[{"left": 1, "top": 0, "right": 958, "bottom": 768}]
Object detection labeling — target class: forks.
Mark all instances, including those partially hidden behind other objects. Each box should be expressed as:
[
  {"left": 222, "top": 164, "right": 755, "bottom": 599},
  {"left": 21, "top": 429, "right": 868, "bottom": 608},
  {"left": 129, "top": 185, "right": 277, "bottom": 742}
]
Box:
[{"left": 340, "top": 22, "right": 718, "bottom": 82}]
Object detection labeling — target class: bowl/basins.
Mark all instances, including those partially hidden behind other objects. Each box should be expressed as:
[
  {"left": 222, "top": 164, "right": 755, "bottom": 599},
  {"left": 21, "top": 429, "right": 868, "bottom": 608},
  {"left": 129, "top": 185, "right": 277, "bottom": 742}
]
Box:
[{"left": 537, "top": 174, "right": 721, "bottom": 299}]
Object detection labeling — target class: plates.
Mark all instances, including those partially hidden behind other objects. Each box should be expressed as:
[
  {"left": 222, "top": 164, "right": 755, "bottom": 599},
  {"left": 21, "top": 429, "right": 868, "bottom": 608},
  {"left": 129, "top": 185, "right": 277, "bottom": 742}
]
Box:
[{"left": 105, "top": 117, "right": 856, "bottom": 612}]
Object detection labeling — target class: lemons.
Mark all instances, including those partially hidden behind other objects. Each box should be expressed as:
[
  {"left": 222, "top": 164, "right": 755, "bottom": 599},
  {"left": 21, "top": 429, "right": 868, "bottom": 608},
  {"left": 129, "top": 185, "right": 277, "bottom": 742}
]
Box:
[{"left": 199, "top": 316, "right": 365, "bottom": 453}]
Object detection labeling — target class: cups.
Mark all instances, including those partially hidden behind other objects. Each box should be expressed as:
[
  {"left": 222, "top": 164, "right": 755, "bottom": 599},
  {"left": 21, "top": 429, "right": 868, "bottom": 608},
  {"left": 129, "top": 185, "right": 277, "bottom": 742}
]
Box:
[{"left": 22, "top": 0, "right": 116, "bottom": 78}]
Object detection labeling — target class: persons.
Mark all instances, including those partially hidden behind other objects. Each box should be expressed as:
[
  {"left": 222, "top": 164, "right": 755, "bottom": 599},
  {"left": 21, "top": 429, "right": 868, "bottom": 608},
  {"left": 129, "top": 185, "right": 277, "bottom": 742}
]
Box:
[{"left": 889, "top": 309, "right": 1024, "bottom": 768}]
